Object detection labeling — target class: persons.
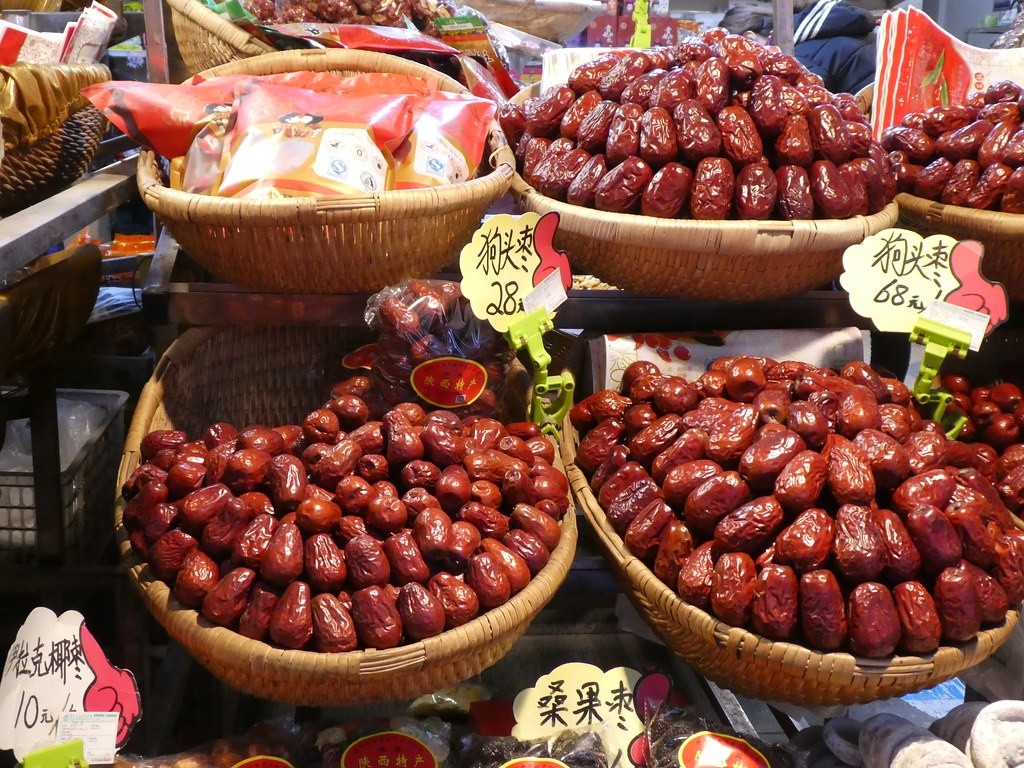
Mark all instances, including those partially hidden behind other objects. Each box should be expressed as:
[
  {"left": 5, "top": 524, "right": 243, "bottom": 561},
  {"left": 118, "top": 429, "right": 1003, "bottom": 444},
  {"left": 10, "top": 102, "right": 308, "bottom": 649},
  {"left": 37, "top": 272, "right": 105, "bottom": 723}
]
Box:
[{"left": 716, "top": 0, "right": 878, "bottom": 125}]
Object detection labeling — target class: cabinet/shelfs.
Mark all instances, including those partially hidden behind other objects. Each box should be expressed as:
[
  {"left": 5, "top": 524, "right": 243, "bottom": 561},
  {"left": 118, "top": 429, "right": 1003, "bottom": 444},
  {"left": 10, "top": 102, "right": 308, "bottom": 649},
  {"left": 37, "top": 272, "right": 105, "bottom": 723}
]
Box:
[{"left": 143, "top": 211, "right": 1024, "bottom": 768}]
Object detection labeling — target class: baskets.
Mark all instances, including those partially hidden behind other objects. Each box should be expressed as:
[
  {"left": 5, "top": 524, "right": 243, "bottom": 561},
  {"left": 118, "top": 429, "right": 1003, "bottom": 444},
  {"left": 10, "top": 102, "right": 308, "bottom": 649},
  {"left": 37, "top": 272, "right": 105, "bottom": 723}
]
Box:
[
  {"left": 136, "top": 48, "right": 516, "bottom": 297},
  {"left": 0, "top": 60, "right": 112, "bottom": 199},
  {"left": 456, "top": 0, "right": 602, "bottom": 41},
  {"left": 162, "top": 0, "right": 277, "bottom": 84},
  {"left": 855, "top": 82, "right": 1024, "bottom": 301},
  {"left": 559, "top": 395, "right": 1024, "bottom": 707},
  {"left": 115, "top": 328, "right": 579, "bottom": 706},
  {"left": 508, "top": 80, "right": 898, "bottom": 300},
  {"left": 1, "top": 243, "right": 102, "bottom": 378}
]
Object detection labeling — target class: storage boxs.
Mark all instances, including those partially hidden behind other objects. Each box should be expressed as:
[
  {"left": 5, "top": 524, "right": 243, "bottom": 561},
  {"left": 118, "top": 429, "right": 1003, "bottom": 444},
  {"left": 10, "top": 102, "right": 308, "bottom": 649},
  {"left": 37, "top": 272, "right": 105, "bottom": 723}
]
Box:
[{"left": 0, "top": 387, "right": 131, "bottom": 567}]
